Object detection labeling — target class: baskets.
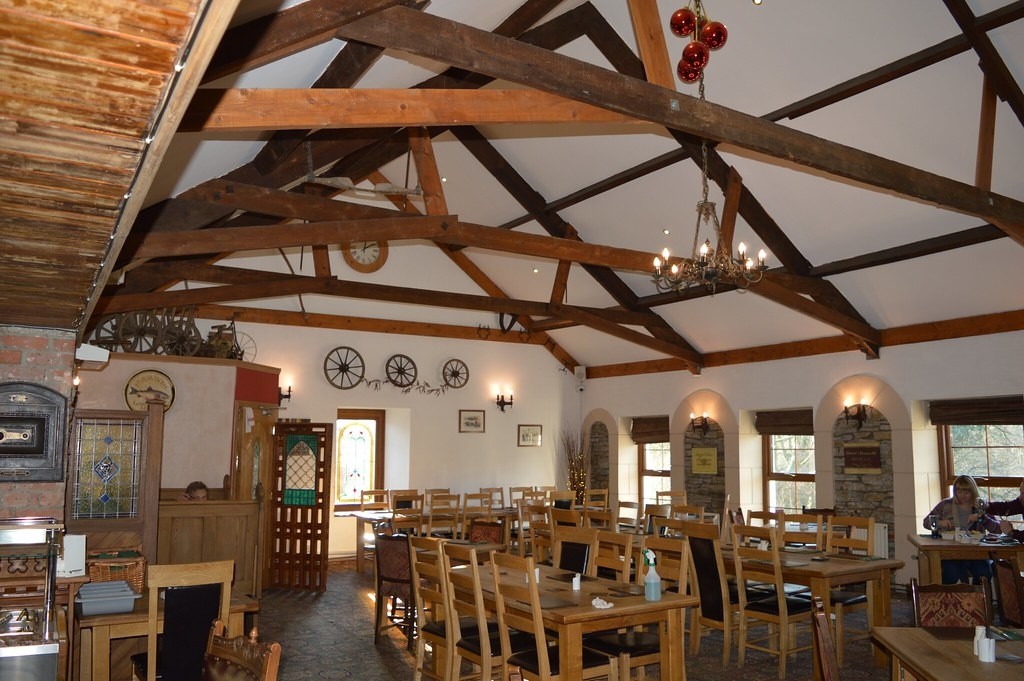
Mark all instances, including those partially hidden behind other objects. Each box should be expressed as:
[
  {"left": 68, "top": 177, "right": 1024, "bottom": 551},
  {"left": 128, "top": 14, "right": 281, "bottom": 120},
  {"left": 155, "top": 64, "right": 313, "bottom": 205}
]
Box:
[{"left": 85, "top": 547, "right": 146, "bottom": 594}]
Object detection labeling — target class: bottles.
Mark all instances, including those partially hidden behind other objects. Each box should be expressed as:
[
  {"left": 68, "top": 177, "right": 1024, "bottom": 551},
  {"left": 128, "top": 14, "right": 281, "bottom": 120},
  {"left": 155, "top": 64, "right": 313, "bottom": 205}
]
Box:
[
  {"left": 642, "top": 549, "right": 661, "bottom": 601},
  {"left": 955, "top": 527, "right": 961, "bottom": 541},
  {"left": 973, "top": 626, "right": 986, "bottom": 656}
]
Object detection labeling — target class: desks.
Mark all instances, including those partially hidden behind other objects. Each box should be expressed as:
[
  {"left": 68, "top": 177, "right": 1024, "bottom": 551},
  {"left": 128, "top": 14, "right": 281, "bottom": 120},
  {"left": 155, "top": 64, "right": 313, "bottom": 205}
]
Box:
[
  {"left": 872, "top": 625, "right": 1024, "bottom": 681},
  {"left": 598, "top": 530, "right": 662, "bottom": 582},
  {"left": 73, "top": 583, "right": 262, "bottom": 681},
  {"left": 348, "top": 507, "right": 529, "bottom": 574},
  {"left": 906, "top": 532, "right": 1024, "bottom": 632},
  {"left": 421, "top": 558, "right": 700, "bottom": 680},
  {"left": 719, "top": 542, "right": 906, "bottom": 668}
]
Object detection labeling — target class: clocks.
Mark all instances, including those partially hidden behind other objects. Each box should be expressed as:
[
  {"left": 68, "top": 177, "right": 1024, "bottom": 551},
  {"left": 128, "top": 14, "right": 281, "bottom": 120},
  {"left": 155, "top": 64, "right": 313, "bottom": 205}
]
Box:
[{"left": 343, "top": 237, "right": 389, "bottom": 272}]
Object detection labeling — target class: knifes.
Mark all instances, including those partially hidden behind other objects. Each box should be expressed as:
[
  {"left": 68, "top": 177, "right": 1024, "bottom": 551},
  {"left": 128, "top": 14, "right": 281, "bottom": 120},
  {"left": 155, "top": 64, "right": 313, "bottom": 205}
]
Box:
[{"left": 989, "top": 625, "right": 1013, "bottom": 640}]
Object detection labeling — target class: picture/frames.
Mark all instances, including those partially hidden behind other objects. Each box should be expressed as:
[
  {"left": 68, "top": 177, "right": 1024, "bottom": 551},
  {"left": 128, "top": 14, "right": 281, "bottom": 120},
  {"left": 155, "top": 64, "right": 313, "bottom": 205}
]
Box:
[
  {"left": 457, "top": 409, "right": 485, "bottom": 434},
  {"left": 518, "top": 423, "right": 543, "bottom": 446}
]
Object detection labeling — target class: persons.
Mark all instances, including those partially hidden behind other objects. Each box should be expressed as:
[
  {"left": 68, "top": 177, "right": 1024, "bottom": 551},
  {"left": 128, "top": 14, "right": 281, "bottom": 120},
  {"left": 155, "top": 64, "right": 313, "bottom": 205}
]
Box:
[
  {"left": 924, "top": 475, "right": 1002, "bottom": 586},
  {"left": 177, "top": 481, "right": 208, "bottom": 500},
  {"left": 986, "top": 479, "right": 1024, "bottom": 543}
]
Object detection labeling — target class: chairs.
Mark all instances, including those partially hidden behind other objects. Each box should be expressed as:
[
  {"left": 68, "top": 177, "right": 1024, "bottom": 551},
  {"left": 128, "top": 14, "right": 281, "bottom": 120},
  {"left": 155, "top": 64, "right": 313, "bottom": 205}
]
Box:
[
  {"left": 204, "top": 619, "right": 282, "bottom": 681},
  {"left": 333, "top": 480, "right": 905, "bottom": 681},
  {"left": 130, "top": 558, "right": 235, "bottom": 681},
  {"left": 989, "top": 551, "right": 1024, "bottom": 629},
  {"left": 910, "top": 575, "right": 993, "bottom": 637}
]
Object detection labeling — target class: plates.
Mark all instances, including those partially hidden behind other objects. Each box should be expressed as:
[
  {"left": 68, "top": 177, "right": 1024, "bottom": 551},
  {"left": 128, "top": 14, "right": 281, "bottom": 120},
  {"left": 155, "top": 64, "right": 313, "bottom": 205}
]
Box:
[{"left": 984, "top": 540, "right": 1002, "bottom": 544}]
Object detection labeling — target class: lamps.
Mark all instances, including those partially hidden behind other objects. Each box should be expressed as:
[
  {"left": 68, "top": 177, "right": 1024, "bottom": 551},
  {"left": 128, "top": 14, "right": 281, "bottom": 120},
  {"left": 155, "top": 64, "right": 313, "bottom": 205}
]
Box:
[
  {"left": 690, "top": 409, "right": 710, "bottom": 437},
  {"left": 842, "top": 395, "right": 869, "bottom": 430},
  {"left": 275, "top": 372, "right": 295, "bottom": 405},
  {"left": 650, "top": 146, "right": 767, "bottom": 296},
  {"left": 491, "top": 383, "right": 515, "bottom": 410}
]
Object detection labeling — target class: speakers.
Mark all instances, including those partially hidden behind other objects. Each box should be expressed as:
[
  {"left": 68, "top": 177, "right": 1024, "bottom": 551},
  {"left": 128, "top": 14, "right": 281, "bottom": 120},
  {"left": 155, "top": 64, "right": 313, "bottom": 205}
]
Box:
[{"left": 575, "top": 366, "right": 587, "bottom": 381}]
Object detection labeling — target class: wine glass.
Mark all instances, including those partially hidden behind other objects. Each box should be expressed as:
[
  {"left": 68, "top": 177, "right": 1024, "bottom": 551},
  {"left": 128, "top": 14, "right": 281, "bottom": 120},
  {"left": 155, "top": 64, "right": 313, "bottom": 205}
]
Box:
[{"left": 929, "top": 514, "right": 939, "bottom": 539}]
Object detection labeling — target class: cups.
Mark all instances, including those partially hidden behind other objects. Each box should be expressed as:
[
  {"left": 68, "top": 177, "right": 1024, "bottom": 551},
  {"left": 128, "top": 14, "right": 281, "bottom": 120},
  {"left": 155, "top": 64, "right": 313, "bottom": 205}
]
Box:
[
  {"left": 977, "top": 639, "right": 995, "bottom": 662},
  {"left": 1002, "top": 536, "right": 1014, "bottom": 543},
  {"left": 984, "top": 536, "right": 998, "bottom": 541},
  {"left": 976, "top": 498, "right": 987, "bottom": 514}
]
